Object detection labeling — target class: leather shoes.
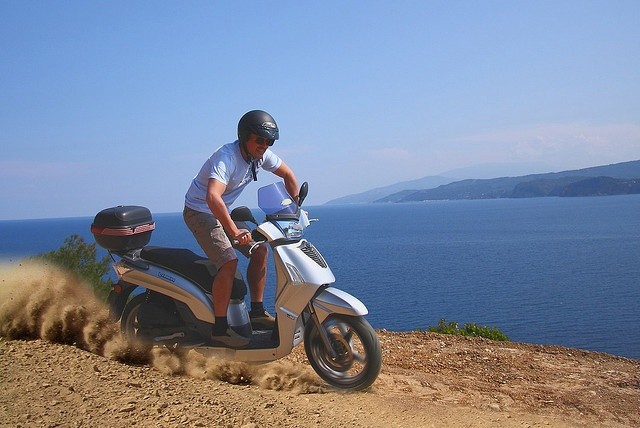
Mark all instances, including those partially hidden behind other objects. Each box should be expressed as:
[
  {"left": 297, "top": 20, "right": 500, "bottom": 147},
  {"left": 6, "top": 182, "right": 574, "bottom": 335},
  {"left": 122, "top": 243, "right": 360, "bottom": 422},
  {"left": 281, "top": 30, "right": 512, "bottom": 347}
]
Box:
[
  {"left": 248, "top": 311, "right": 276, "bottom": 330},
  {"left": 209, "top": 324, "right": 251, "bottom": 349}
]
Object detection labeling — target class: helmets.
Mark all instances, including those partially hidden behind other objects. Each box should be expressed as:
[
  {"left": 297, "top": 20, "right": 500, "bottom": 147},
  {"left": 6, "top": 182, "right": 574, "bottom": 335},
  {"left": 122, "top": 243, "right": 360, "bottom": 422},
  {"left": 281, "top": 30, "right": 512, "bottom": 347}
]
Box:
[{"left": 238, "top": 110, "right": 279, "bottom": 142}]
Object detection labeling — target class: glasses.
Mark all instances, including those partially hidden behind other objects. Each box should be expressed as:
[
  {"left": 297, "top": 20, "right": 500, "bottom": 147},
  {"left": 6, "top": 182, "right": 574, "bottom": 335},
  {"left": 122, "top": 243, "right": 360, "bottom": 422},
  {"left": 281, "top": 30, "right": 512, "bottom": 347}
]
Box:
[{"left": 255, "top": 138, "right": 275, "bottom": 146}]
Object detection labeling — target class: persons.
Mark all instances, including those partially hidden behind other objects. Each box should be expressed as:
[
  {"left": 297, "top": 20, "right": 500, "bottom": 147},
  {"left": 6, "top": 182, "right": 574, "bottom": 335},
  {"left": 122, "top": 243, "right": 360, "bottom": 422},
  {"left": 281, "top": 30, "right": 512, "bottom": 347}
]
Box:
[{"left": 183, "top": 111, "right": 309, "bottom": 349}]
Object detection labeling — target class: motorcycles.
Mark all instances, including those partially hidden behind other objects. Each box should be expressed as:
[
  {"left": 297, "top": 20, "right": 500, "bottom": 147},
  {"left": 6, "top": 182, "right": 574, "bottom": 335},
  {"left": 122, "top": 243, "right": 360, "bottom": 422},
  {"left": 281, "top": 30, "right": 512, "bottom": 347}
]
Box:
[{"left": 91, "top": 181, "right": 384, "bottom": 391}]
